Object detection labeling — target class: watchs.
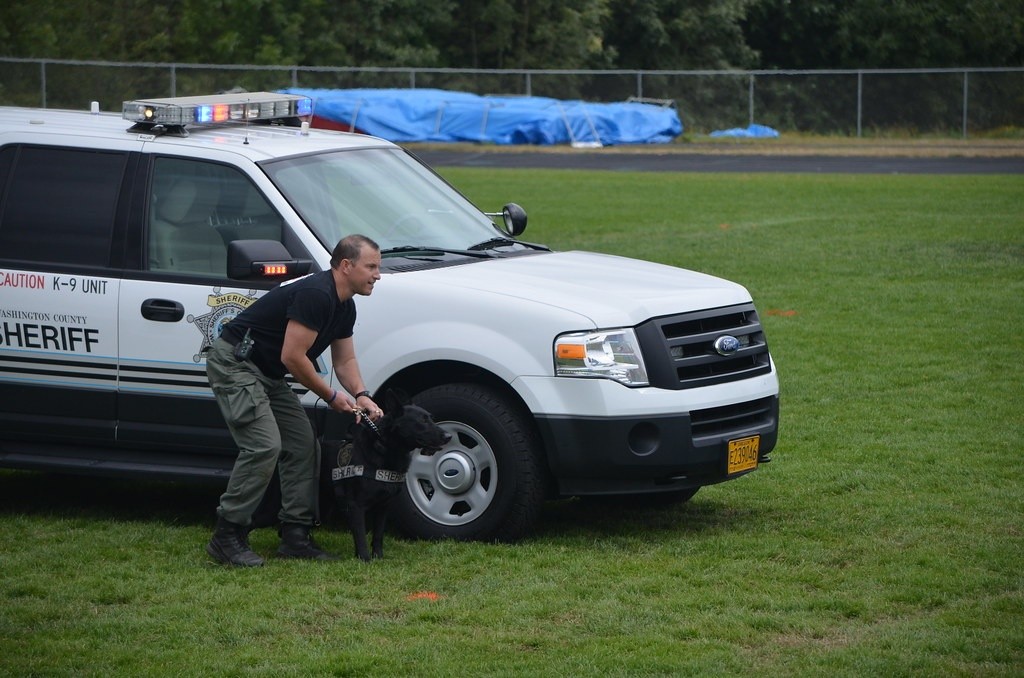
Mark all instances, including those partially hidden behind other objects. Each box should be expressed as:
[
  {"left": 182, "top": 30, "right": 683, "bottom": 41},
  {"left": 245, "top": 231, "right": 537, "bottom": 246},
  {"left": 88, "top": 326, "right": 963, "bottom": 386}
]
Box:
[{"left": 355, "top": 391, "right": 372, "bottom": 399}]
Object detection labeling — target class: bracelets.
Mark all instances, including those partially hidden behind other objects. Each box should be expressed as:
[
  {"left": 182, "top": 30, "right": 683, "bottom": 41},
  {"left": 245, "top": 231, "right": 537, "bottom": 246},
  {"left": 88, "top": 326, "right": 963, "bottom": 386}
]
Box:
[{"left": 326, "top": 389, "right": 337, "bottom": 404}]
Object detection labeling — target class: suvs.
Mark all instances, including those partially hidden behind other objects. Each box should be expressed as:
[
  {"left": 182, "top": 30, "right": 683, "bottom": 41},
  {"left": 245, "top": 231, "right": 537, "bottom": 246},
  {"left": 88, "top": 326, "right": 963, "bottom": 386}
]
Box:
[{"left": 0, "top": 90, "right": 780, "bottom": 545}]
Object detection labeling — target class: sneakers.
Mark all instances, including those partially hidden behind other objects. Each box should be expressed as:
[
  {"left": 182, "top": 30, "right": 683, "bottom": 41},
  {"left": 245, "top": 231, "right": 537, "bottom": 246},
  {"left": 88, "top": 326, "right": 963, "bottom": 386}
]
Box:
[
  {"left": 207, "top": 523, "right": 264, "bottom": 568},
  {"left": 277, "top": 533, "right": 325, "bottom": 560}
]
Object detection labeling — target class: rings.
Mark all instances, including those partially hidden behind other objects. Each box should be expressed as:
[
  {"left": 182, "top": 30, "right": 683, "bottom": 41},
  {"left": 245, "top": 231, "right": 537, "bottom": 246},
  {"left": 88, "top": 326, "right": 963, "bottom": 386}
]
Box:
[{"left": 376, "top": 411, "right": 380, "bottom": 413}]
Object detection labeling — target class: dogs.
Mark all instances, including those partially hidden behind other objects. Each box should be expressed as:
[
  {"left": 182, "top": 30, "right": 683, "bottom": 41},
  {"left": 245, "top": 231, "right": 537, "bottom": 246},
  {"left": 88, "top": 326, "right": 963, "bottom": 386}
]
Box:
[{"left": 242, "top": 401, "right": 453, "bottom": 562}]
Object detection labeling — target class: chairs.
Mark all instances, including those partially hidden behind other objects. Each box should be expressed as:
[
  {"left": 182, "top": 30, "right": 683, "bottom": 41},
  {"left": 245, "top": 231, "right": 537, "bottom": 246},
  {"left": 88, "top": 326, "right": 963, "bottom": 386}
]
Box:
[
  {"left": 153, "top": 176, "right": 230, "bottom": 277},
  {"left": 233, "top": 182, "right": 283, "bottom": 244}
]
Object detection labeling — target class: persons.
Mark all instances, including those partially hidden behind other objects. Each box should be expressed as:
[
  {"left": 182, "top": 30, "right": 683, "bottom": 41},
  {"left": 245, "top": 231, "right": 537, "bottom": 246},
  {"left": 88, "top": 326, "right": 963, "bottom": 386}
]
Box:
[{"left": 205, "top": 234, "right": 384, "bottom": 569}]
